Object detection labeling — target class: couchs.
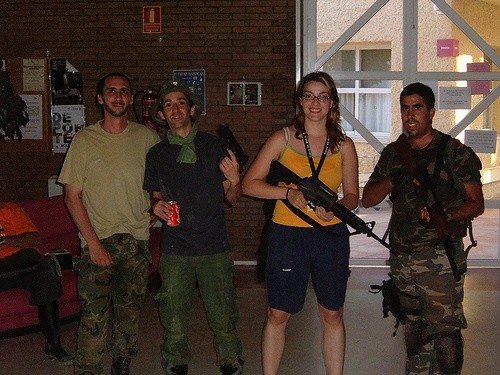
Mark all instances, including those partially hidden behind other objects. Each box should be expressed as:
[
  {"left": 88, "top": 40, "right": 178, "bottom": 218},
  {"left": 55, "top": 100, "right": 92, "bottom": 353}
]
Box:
[{"left": 0, "top": 194, "right": 163, "bottom": 340}]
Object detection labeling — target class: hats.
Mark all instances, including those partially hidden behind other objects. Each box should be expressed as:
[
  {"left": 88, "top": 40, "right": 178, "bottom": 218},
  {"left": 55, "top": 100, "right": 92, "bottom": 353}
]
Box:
[{"left": 150, "top": 81, "right": 202, "bottom": 126}]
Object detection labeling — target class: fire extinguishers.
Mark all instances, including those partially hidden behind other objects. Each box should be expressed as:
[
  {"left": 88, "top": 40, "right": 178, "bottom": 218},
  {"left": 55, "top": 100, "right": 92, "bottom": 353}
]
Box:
[{"left": 132, "top": 84, "right": 162, "bottom": 136}]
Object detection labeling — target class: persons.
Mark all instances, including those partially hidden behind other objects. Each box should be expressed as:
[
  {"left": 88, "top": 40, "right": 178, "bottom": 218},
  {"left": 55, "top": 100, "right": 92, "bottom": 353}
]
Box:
[
  {"left": 361, "top": 83, "right": 484, "bottom": 375},
  {"left": 55, "top": 71, "right": 160, "bottom": 375},
  {"left": 240, "top": 71, "right": 359, "bottom": 375},
  {"left": 142, "top": 79, "right": 245, "bottom": 375},
  {"left": 0, "top": 201, "right": 77, "bottom": 367}
]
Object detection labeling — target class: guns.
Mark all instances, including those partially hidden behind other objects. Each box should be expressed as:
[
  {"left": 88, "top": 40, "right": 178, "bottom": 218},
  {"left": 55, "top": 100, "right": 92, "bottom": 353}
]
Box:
[
  {"left": 216, "top": 122, "right": 244, "bottom": 164},
  {"left": 389, "top": 133, "right": 463, "bottom": 282},
  {"left": 266, "top": 159, "right": 401, "bottom": 258}
]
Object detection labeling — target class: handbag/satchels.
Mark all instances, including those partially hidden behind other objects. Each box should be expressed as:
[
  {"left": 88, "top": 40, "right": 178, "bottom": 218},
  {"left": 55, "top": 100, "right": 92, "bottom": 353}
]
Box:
[{"left": 44, "top": 248, "right": 76, "bottom": 276}]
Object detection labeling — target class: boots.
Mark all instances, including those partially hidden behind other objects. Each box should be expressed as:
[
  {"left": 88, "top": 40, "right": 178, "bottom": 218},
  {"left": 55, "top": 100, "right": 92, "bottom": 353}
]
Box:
[
  {"left": 166, "top": 363, "right": 188, "bottom": 375},
  {"left": 220, "top": 359, "right": 243, "bottom": 375},
  {"left": 37, "top": 300, "right": 74, "bottom": 366},
  {"left": 111, "top": 357, "right": 131, "bottom": 375}
]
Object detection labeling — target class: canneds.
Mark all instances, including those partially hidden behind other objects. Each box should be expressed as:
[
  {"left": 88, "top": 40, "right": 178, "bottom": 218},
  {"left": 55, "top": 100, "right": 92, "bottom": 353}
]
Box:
[{"left": 165, "top": 201, "right": 180, "bottom": 226}]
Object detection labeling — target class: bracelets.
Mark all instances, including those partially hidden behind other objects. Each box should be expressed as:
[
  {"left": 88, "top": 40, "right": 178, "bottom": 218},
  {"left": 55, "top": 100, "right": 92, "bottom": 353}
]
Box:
[
  {"left": 0, "top": 236, "right": 6, "bottom": 247},
  {"left": 286, "top": 187, "right": 293, "bottom": 200}
]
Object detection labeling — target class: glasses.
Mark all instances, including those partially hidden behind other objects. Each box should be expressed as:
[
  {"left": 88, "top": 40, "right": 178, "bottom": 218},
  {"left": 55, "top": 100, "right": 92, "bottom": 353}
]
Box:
[{"left": 302, "top": 90, "right": 332, "bottom": 102}]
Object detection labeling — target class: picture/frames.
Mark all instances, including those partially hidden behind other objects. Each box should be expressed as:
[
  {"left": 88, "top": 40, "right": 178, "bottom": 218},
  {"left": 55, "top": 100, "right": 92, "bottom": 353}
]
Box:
[{"left": 227, "top": 82, "right": 262, "bottom": 107}]
